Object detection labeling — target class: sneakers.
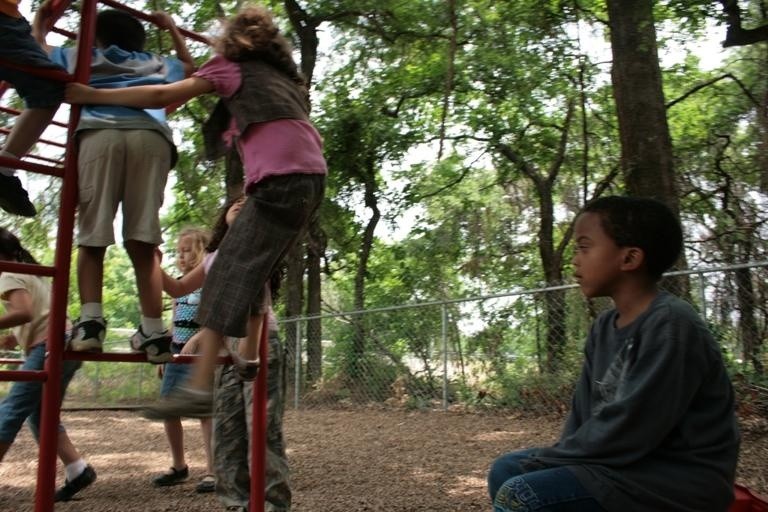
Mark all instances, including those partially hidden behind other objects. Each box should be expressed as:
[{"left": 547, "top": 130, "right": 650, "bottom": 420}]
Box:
[
  {"left": 55, "top": 465, "right": 96, "bottom": 501},
  {"left": 130, "top": 325, "right": 175, "bottom": 363},
  {"left": 196, "top": 474, "right": 216, "bottom": 492},
  {"left": 68, "top": 315, "right": 107, "bottom": 353},
  {"left": 144, "top": 386, "right": 214, "bottom": 418},
  {"left": 154, "top": 466, "right": 188, "bottom": 486},
  {"left": 0, "top": 172, "right": 36, "bottom": 217},
  {"left": 225, "top": 336, "right": 261, "bottom": 381}
]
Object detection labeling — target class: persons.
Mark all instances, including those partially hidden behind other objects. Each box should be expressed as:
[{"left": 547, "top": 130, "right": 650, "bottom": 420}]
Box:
[
  {"left": 146, "top": 224, "right": 221, "bottom": 494},
  {"left": 1, "top": 226, "right": 97, "bottom": 507},
  {"left": 0, "top": 0, "right": 71, "bottom": 218},
  {"left": 61, "top": 1, "right": 332, "bottom": 424},
  {"left": 484, "top": 189, "right": 742, "bottom": 509},
  {"left": 155, "top": 190, "right": 294, "bottom": 510},
  {"left": 30, "top": 0, "right": 197, "bottom": 366}
]
